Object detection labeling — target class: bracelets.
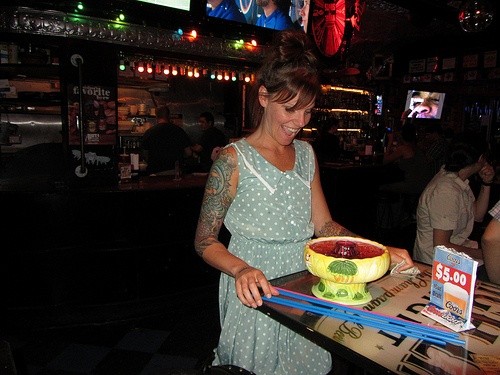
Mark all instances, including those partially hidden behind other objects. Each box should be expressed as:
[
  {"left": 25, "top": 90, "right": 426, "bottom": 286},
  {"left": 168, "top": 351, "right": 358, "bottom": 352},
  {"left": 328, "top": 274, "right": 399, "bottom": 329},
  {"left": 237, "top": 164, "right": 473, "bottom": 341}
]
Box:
[{"left": 482, "top": 181, "right": 493, "bottom": 186}]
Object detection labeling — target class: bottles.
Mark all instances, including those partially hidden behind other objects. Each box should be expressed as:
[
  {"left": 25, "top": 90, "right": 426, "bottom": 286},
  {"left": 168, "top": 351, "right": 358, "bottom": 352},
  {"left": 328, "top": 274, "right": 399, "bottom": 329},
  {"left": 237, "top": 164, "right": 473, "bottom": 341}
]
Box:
[
  {"left": 365, "top": 136, "right": 373, "bottom": 156},
  {"left": 317, "top": 92, "right": 370, "bottom": 110},
  {"left": 118, "top": 145, "right": 129, "bottom": 172},
  {"left": 87, "top": 103, "right": 97, "bottom": 134},
  {"left": 316, "top": 112, "right": 361, "bottom": 129},
  {"left": 97, "top": 103, "right": 108, "bottom": 133},
  {"left": 130, "top": 139, "right": 139, "bottom": 173}
]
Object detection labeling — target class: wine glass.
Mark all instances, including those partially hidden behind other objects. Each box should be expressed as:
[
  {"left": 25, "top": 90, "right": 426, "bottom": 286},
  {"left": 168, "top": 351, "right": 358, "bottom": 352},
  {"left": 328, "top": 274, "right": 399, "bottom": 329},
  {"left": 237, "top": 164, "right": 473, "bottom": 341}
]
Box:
[
  {"left": 168, "top": 32, "right": 273, "bottom": 60},
  {"left": 304, "top": 236, "right": 391, "bottom": 305},
  {"left": 6, "top": 10, "right": 97, "bottom": 40},
  {"left": 97, "top": 20, "right": 168, "bottom": 49}
]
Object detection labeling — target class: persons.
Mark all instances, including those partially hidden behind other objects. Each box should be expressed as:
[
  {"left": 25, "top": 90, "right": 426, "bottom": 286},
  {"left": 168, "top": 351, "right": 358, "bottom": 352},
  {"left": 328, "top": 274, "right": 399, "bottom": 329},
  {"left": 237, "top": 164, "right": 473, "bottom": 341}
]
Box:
[
  {"left": 413, "top": 130, "right": 500, "bottom": 285},
  {"left": 192, "top": 112, "right": 228, "bottom": 171},
  {"left": 444, "top": 127, "right": 455, "bottom": 146},
  {"left": 408, "top": 90, "right": 445, "bottom": 119},
  {"left": 206, "top": 0, "right": 296, "bottom": 30},
  {"left": 374, "top": 123, "right": 455, "bottom": 228},
  {"left": 300, "top": 0, "right": 310, "bottom": 32},
  {"left": 313, "top": 117, "right": 349, "bottom": 161},
  {"left": 139, "top": 103, "right": 192, "bottom": 172},
  {"left": 195, "top": 28, "right": 419, "bottom": 375}
]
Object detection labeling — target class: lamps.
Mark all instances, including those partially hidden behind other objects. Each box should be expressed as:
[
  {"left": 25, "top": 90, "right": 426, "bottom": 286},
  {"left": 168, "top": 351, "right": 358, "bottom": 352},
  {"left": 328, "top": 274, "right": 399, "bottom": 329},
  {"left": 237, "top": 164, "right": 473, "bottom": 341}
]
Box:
[{"left": 119, "top": 59, "right": 257, "bottom": 85}]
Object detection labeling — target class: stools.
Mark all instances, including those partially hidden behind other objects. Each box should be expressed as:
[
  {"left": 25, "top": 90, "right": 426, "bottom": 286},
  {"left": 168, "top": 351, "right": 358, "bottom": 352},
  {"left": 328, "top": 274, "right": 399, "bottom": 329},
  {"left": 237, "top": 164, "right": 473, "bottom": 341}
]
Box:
[{"left": 364, "top": 188, "right": 420, "bottom": 243}]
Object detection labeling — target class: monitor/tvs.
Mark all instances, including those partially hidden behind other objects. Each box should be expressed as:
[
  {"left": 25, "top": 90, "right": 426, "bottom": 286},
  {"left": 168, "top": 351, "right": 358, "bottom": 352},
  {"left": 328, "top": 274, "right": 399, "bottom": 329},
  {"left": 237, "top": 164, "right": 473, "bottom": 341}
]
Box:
[
  {"left": 107, "top": 0, "right": 314, "bottom": 45},
  {"left": 403, "top": 89, "right": 446, "bottom": 121}
]
